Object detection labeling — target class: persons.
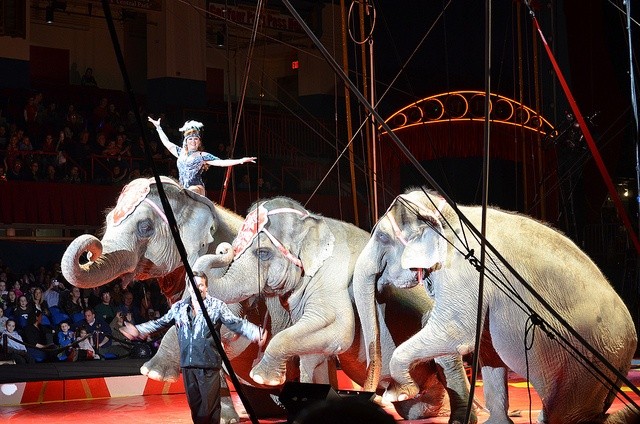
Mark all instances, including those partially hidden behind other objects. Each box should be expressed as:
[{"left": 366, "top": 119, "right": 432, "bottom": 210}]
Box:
[
  {"left": 111, "top": 312, "right": 134, "bottom": 349},
  {"left": 257, "top": 178, "right": 268, "bottom": 191},
  {"left": 0, "top": 282, "right": 9, "bottom": 297},
  {"left": 13, "top": 296, "right": 33, "bottom": 328},
  {"left": 43, "top": 280, "right": 65, "bottom": 324},
  {"left": 92, "top": 287, "right": 120, "bottom": 324},
  {"left": 1, "top": 319, "right": 35, "bottom": 365},
  {"left": 121, "top": 271, "right": 268, "bottom": 424},
  {"left": 27, "top": 289, "right": 52, "bottom": 323},
  {"left": 138, "top": 291, "right": 153, "bottom": 323},
  {"left": 52, "top": 262, "right": 60, "bottom": 277},
  {"left": 112, "top": 284, "right": 121, "bottom": 305},
  {"left": 240, "top": 174, "right": 257, "bottom": 190},
  {"left": 141, "top": 307, "right": 163, "bottom": 351},
  {"left": 82, "top": 309, "right": 112, "bottom": 356},
  {"left": 11, "top": 279, "right": 24, "bottom": 297},
  {"left": 57, "top": 322, "right": 76, "bottom": 362},
  {"left": 5, "top": 290, "right": 17, "bottom": 309},
  {"left": 1, "top": 94, "right": 177, "bottom": 182},
  {"left": 28, "top": 285, "right": 39, "bottom": 294},
  {"left": 26, "top": 309, "right": 45, "bottom": 348},
  {"left": 72, "top": 327, "right": 100, "bottom": 359},
  {"left": 1, "top": 308, "right": 8, "bottom": 332},
  {"left": 64, "top": 286, "right": 87, "bottom": 314},
  {"left": 147, "top": 115, "right": 258, "bottom": 199},
  {"left": 215, "top": 141, "right": 235, "bottom": 157},
  {"left": 0, "top": 271, "right": 8, "bottom": 283},
  {"left": 114, "top": 291, "right": 140, "bottom": 321}
]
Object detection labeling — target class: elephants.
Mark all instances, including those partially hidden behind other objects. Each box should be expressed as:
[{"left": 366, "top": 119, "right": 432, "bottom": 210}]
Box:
[
  {"left": 351, "top": 186, "right": 640, "bottom": 424},
  {"left": 191, "top": 194, "right": 479, "bottom": 424},
  {"left": 60, "top": 175, "right": 338, "bottom": 424}
]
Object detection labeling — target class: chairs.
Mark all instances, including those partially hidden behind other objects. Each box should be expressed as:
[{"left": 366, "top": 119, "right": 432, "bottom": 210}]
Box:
[{"left": 20, "top": 307, "right": 116, "bottom": 362}]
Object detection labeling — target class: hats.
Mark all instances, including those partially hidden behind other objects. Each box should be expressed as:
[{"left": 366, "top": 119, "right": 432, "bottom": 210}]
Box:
[{"left": 183, "top": 128, "right": 200, "bottom": 139}]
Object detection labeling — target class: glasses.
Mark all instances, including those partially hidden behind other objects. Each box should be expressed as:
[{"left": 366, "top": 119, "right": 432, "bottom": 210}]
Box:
[
  {"left": 73, "top": 290, "right": 80, "bottom": 292},
  {"left": 103, "top": 293, "right": 110, "bottom": 296}
]
